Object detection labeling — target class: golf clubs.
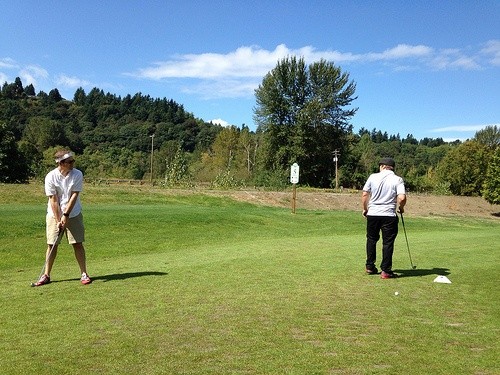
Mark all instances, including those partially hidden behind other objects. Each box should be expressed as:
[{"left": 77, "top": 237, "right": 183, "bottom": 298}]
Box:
[
  {"left": 31, "top": 227, "right": 63, "bottom": 287},
  {"left": 400, "top": 212, "right": 416, "bottom": 269}
]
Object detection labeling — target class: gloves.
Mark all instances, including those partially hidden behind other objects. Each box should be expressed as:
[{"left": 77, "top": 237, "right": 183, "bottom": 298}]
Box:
[{"left": 362, "top": 210, "right": 368, "bottom": 218}]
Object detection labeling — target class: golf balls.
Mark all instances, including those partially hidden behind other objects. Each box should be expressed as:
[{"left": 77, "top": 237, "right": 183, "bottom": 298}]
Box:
[{"left": 394, "top": 291, "right": 399, "bottom": 297}]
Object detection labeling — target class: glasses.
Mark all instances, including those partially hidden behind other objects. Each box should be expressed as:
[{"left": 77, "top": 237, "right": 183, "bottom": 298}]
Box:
[{"left": 67, "top": 159, "right": 75, "bottom": 163}]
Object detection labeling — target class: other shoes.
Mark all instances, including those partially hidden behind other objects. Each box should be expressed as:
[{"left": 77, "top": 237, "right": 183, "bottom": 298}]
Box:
[
  {"left": 366, "top": 266, "right": 378, "bottom": 273},
  {"left": 81, "top": 273, "right": 90, "bottom": 284},
  {"left": 380, "top": 271, "right": 398, "bottom": 278},
  {"left": 34, "top": 274, "right": 50, "bottom": 286}
]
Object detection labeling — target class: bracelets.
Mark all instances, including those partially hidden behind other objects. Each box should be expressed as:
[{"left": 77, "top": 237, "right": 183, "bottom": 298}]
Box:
[{"left": 63, "top": 213, "right": 69, "bottom": 217}]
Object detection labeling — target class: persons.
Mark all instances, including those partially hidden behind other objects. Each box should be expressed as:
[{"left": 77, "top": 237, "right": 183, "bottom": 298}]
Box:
[
  {"left": 361, "top": 158, "right": 406, "bottom": 279},
  {"left": 34, "top": 149, "right": 91, "bottom": 285}
]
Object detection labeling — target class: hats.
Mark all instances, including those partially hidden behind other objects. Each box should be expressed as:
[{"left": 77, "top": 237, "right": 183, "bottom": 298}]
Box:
[{"left": 379, "top": 158, "right": 395, "bottom": 166}]
